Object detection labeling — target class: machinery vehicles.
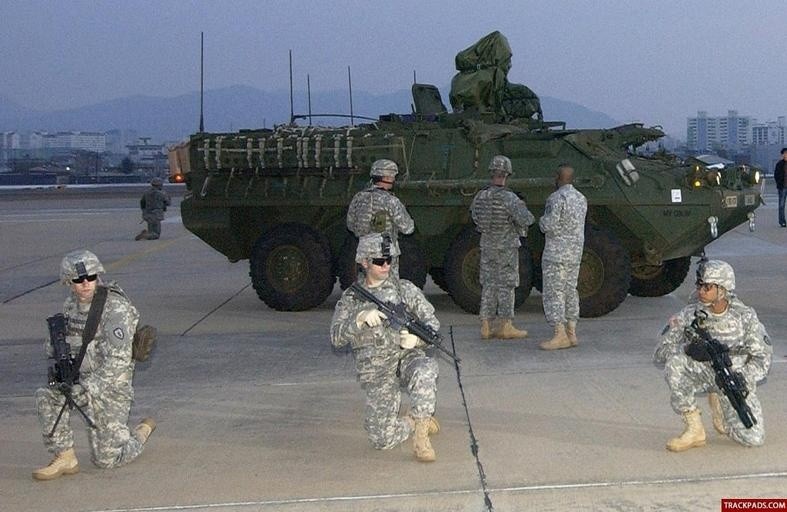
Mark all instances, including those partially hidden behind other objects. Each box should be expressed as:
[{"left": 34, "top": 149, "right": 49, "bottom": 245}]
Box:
[{"left": 166, "top": 27, "right": 766, "bottom": 319}]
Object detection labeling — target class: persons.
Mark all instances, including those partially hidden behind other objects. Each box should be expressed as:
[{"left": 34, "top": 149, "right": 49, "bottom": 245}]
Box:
[
  {"left": 330, "top": 232, "right": 440, "bottom": 461},
  {"left": 652, "top": 260, "right": 774, "bottom": 451},
  {"left": 135, "top": 177, "right": 171, "bottom": 240},
  {"left": 471, "top": 156, "right": 535, "bottom": 340},
  {"left": 539, "top": 165, "right": 588, "bottom": 351},
  {"left": 32, "top": 250, "right": 156, "bottom": 480},
  {"left": 774, "top": 148, "right": 787, "bottom": 227},
  {"left": 355, "top": 159, "right": 414, "bottom": 281}
]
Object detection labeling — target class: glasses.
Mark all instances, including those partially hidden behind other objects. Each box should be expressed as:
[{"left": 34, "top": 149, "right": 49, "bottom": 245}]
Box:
[
  {"left": 72, "top": 274, "right": 97, "bottom": 283},
  {"left": 373, "top": 256, "right": 392, "bottom": 266}
]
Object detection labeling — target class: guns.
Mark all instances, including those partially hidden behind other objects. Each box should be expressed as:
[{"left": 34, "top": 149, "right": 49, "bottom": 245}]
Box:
[
  {"left": 344, "top": 281, "right": 462, "bottom": 364},
  {"left": 684, "top": 324, "right": 758, "bottom": 428},
  {"left": 48, "top": 314, "right": 98, "bottom": 437}
]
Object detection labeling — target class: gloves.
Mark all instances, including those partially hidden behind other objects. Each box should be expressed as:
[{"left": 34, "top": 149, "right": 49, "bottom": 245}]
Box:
[
  {"left": 400, "top": 330, "right": 417, "bottom": 349},
  {"left": 356, "top": 309, "right": 387, "bottom": 329}
]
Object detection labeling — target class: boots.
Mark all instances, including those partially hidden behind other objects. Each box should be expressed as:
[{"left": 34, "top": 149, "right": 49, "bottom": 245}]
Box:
[
  {"left": 32, "top": 448, "right": 80, "bottom": 480},
  {"left": 133, "top": 419, "right": 156, "bottom": 445},
  {"left": 709, "top": 393, "right": 726, "bottom": 433},
  {"left": 481, "top": 319, "right": 527, "bottom": 338},
  {"left": 135, "top": 229, "right": 151, "bottom": 240},
  {"left": 406, "top": 411, "right": 440, "bottom": 461},
  {"left": 539, "top": 321, "right": 578, "bottom": 350},
  {"left": 666, "top": 410, "right": 705, "bottom": 452}
]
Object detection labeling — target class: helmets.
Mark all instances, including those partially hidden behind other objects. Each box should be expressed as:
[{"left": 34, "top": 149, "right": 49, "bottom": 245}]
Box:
[
  {"left": 697, "top": 260, "right": 736, "bottom": 291},
  {"left": 488, "top": 155, "right": 512, "bottom": 174},
  {"left": 355, "top": 232, "right": 395, "bottom": 263},
  {"left": 60, "top": 250, "right": 104, "bottom": 283},
  {"left": 151, "top": 177, "right": 163, "bottom": 186},
  {"left": 369, "top": 159, "right": 398, "bottom": 176}
]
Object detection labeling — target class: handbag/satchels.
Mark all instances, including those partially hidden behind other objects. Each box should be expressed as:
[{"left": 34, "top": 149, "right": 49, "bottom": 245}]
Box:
[{"left": 133, "top": 326, "right": 157, "bottom": 361}]
